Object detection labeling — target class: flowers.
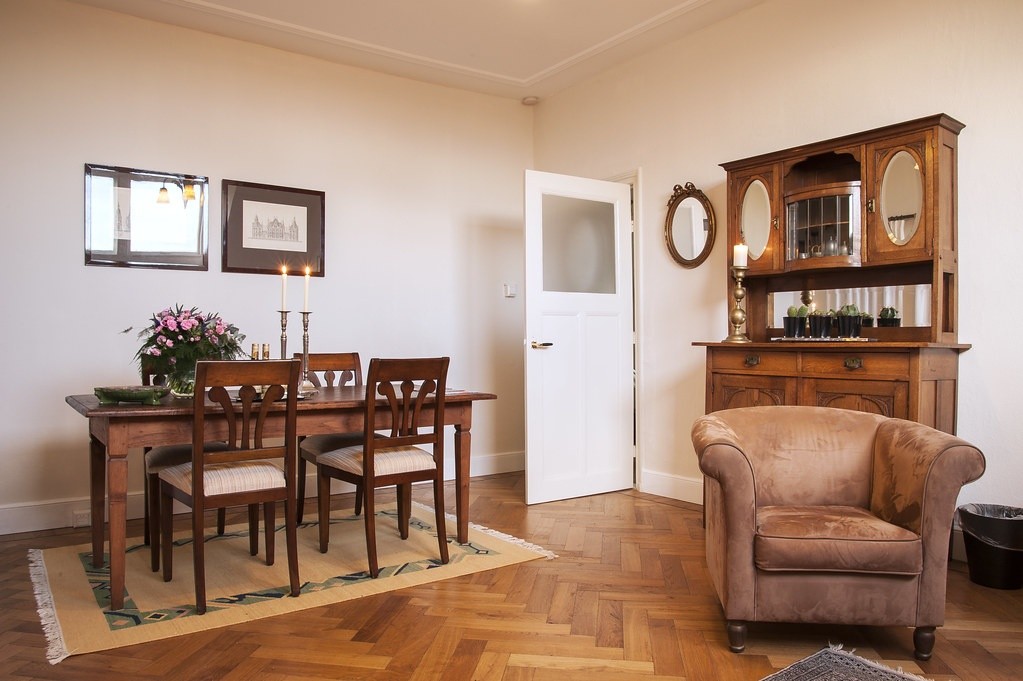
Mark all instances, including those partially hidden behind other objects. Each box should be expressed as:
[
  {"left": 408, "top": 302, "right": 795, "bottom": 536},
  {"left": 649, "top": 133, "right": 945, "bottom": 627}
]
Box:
[{"left": 120, "top": 303, "right": 253, "bottom": 394}]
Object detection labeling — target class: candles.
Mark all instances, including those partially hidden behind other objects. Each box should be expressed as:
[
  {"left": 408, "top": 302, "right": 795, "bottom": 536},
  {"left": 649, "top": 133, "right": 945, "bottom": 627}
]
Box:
[
  {"left": 281, "top": 266, "right": 287, "bottom": 310},
  {"left": 304, "top": 267, "right": 310, "bottom": 312},
  {"left": 733, "top": 243, "right": 748, "bottom": 266}
]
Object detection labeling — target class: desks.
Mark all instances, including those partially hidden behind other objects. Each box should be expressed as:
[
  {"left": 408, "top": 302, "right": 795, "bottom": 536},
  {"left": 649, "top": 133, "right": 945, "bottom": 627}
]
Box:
[{"left": 65, "top": 384, "right": 498, "bottom": 611}]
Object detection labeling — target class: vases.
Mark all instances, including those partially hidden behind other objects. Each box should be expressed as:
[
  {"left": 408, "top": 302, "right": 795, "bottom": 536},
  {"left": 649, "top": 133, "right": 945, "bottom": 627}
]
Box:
[{"left": 165, "top": 370, "right": 196, "bottom": 400}]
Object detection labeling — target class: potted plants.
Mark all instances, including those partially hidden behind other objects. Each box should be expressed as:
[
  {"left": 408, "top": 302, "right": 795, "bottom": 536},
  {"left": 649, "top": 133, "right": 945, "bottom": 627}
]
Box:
[
  {"left": 783, "top": 304, "right": 863, "bottom": 338},
  {"left": 861, "top": 312, "right": 874, "bottom": 327},
  {"left": 877, "top": 307, "right": 901, "bottom": 327}
]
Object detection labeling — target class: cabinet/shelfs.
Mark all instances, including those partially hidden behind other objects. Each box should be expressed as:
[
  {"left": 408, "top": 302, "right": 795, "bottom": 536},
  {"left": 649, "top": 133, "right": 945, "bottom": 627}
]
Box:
[{"left": 691, "top": 113, "right": 967, "bottom": 529}]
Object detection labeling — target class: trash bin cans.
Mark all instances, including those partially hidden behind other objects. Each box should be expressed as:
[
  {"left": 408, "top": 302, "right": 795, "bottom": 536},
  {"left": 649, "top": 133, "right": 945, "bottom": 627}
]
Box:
[{"left": 958, "top": 503, "right": 1023, "bottom": 589}]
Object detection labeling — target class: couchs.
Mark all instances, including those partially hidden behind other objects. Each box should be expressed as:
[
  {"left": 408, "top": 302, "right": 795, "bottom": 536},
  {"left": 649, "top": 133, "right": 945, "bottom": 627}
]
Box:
[{"left": 690, "top": 406, "right": 986, "bottom": 662}]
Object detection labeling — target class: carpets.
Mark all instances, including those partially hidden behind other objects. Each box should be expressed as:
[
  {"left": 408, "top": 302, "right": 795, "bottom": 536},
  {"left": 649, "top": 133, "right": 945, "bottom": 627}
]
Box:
[
  {"left": 27, "top": 500, "right": 560, "bottom": 665},
  {"left": 758, "top": 641, "right": 935, "bottom": 681}
]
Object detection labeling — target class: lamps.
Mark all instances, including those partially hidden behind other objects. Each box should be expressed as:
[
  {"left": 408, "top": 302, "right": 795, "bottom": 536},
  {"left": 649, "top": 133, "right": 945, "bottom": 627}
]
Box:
[{"left": 158, "top": 178, "right": 196, "bottom": 208}]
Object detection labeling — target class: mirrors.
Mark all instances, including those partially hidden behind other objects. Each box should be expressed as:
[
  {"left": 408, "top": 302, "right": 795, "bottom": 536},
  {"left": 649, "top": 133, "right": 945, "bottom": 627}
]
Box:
[{"left": 664, "top": 182, "right": 715, "bottom": 269}]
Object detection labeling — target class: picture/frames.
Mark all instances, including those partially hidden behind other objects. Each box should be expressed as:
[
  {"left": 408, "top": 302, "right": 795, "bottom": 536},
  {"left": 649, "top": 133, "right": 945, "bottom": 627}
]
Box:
[
  {"left": 222, "top": 179, "right": 325, "bottom": 276},
  {"left": 84, "top": 163, "right": 208, "bottom": 271}
]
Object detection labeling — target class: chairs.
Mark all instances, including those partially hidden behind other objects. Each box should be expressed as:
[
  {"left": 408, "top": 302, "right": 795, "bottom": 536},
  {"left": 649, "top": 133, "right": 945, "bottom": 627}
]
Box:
[{"left": 142, "top": 353, "right": 450, "bottom": 616}]
